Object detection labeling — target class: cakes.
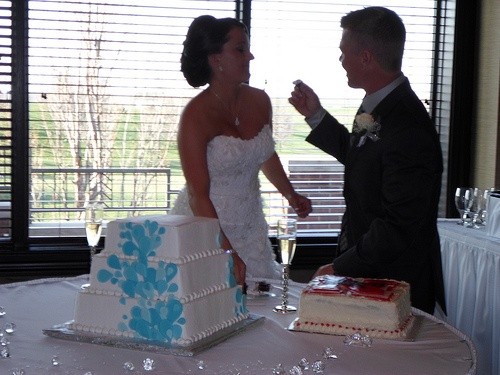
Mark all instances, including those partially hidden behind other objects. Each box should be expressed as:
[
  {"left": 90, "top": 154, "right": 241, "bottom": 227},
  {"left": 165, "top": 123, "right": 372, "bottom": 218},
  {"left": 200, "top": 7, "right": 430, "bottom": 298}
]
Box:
[
  {"left": 294, "top": 273, "right": 414, "bottom": 339},
  {"left": 68, "top": 214, "right": 249, "bottom": 343}
]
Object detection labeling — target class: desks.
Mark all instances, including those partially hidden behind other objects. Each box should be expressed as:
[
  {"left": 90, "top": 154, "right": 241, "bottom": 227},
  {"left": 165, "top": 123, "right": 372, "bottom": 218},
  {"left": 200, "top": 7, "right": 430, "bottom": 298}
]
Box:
[
  {"left": 434, "top": 218, "right": 500, "bottom": 375},
  {"left": 0, "top": 275, "right": 477, "bottom": 375}
]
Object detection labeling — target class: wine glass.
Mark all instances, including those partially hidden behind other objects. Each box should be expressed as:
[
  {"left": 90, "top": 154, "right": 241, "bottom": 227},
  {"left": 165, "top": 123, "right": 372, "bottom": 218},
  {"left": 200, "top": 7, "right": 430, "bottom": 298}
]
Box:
[
  {"left": 272, "top": 218, "right": 297, "bottom": 314},
  {"left": 455, "top": 188, "right": 500, "bottom": 229},
  {"left": 80, "top": 204, "right": 104, "bottom": 290}
]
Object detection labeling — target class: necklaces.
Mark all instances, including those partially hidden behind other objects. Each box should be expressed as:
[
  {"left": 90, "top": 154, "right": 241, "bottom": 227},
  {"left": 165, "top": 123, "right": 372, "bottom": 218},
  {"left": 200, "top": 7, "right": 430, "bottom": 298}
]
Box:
[{"left": 216, "top": 93, "right": 240, "bottom": 125}]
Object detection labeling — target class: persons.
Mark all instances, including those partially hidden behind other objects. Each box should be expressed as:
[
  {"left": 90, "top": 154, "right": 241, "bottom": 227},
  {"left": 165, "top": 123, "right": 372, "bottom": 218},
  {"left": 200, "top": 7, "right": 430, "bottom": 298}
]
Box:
[
  {"left": 289, "top": 6, "right": 447, "bottom": 314},
  {"left": 171, "top": 15, "right": 312, "bottom": 284}
]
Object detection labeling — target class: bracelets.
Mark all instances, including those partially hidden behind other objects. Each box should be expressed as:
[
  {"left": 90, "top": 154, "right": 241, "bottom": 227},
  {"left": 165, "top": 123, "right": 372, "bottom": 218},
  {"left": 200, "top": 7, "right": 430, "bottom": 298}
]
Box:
[{"left": 225, "top": 249, "right": 238, "bottom": 254}]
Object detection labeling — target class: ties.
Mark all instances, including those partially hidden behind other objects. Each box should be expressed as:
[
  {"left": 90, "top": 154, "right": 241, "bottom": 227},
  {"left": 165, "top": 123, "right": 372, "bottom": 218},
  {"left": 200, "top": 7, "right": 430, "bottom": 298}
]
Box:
[{"left": 350, "top": 104, "right": 365, "bottom": 147}]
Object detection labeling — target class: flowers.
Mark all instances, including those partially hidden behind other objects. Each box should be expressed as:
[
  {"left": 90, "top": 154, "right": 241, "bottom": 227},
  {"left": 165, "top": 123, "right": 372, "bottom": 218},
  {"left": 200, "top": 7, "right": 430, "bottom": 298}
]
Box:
[{"left": 353, "top": 113, "right": 373, "bottom": 132}]
